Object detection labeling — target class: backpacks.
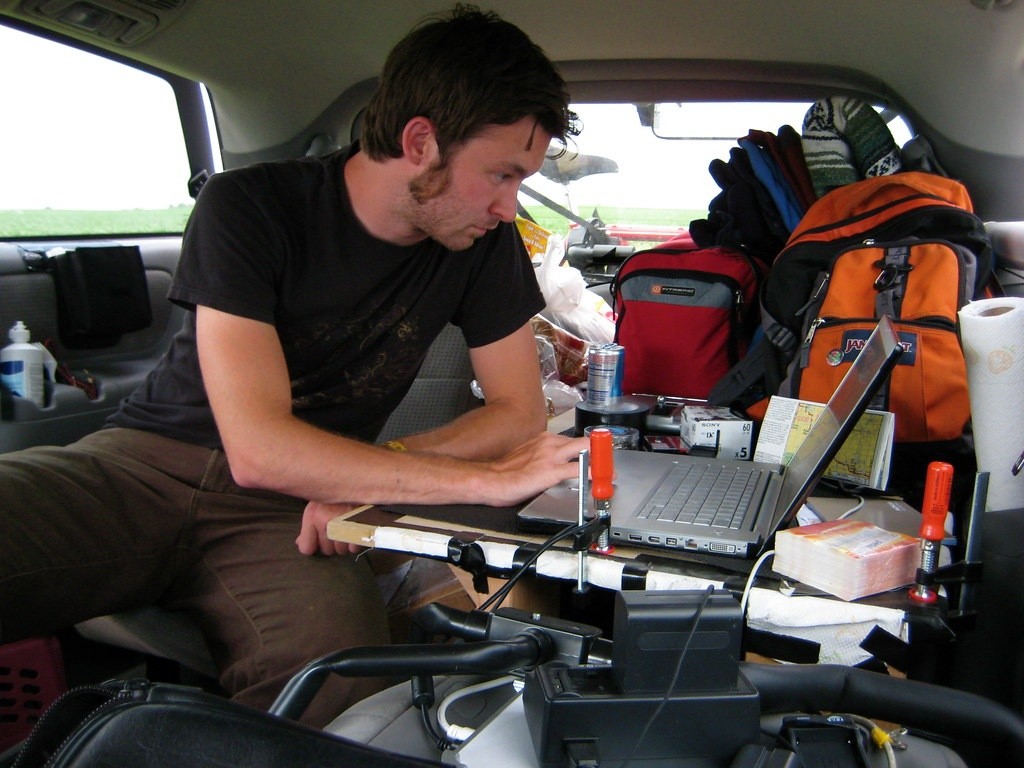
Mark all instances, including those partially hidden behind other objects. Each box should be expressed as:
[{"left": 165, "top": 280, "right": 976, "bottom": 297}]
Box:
[
  {"left": 609, "top": 228, "right": 771, "bottom": 413},
  {"left": 753, "top": 169, "right": 992, "bottom": 445}
]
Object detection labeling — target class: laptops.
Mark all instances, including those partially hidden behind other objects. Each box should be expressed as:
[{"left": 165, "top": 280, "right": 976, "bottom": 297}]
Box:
[{"left": 518, "top": 312, "right": 904, "bottom": 558}]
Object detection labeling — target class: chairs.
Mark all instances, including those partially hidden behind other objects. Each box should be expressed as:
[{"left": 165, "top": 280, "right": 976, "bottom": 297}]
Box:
[{"left": 73, "top": 318, "right": 481, "bottom": 699}]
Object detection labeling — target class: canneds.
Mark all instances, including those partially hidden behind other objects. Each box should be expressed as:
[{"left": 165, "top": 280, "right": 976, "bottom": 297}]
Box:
[{"left": 587, "top": 343, "right": 624, "bottom": 405}]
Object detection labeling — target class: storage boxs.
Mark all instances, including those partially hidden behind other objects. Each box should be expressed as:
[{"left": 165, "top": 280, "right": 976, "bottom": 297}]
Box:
[
  {"left": 642, "top": 434, "right": 691, "bottom": 455},
  {"left": 681, "top": 405, "right": 753, "bottom": 461}
]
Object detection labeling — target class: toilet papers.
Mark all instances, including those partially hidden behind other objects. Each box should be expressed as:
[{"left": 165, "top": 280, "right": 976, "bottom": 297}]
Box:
[{"left": 957, "top": 297, "right": 1024, "bottom": 512}]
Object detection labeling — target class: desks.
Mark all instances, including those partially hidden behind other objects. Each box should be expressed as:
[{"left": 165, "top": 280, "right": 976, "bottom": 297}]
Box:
[{"left": 327, "top": 404, "right": 952, "bottom": 673}]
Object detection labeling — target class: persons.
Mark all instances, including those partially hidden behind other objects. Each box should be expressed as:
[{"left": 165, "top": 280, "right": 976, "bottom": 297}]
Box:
[{"left": 0, "top": 2, "right": 592, "bottom": 734}]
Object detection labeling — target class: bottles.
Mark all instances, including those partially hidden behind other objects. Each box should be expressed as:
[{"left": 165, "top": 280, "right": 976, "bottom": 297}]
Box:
[{"left": 0, "top": 321, "right": 44, "bottom": 409}]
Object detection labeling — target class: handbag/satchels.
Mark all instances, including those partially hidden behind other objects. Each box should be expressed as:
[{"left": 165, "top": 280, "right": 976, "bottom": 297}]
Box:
[
  {"left": 10, "top": 675, "right": 458, "bottom": 768},
  {"left": 532, "top": 262, "right": 619, "bottom": 347}
]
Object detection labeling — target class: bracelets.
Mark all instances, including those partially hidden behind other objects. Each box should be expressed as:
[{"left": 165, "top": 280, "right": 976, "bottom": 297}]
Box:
[{"left": 382, "top": 439, "right": 410, "bottom": 453}]
